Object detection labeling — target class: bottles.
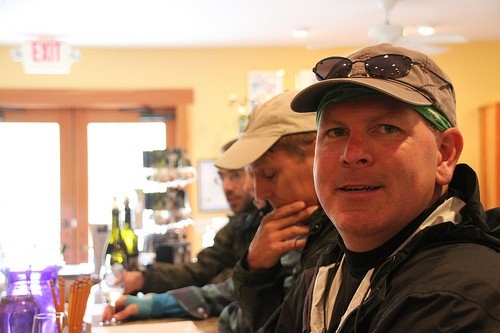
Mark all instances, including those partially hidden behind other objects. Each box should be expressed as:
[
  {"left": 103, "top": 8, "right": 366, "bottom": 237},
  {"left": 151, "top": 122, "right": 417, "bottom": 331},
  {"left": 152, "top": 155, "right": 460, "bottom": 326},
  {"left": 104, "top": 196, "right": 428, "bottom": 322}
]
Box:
[
  {"left": 106, "top": 197, "right": 128, "bottom": 270},
  {"left": 121, "top": 194, "right": 138, "bottom": 257}
]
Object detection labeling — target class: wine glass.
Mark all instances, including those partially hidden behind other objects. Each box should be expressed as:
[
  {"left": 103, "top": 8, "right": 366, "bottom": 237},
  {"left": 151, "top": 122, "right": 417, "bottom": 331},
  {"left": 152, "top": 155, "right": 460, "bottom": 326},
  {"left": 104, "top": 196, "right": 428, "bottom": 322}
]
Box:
[{"left": 99, "top": 264, "right": 126, "bottom": 327}]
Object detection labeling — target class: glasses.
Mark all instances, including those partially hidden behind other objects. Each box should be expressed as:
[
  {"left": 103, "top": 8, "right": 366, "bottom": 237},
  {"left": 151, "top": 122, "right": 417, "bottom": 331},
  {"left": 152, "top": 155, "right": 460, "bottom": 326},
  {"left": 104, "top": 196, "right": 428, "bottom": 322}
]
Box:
[{"left": 312, "top": 54, "right": 453, "bottom": 91}]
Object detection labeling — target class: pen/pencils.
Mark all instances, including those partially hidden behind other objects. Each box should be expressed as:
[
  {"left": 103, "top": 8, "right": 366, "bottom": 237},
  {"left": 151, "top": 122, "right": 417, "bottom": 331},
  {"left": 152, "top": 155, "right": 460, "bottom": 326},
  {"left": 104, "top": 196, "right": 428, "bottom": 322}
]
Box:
[{"left": 47, "top": 277, "right": 93, "bottom": 333}]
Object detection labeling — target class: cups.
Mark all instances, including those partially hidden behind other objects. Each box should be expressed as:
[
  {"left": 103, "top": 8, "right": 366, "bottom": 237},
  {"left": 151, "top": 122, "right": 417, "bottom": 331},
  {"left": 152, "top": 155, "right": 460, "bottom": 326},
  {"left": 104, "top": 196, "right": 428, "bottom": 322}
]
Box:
[
  {"left": 32, "top": 312, "right": 68, "bottom": 333},
  {"left": 63, "top": 322, "right": 90, "bottom": 333}
]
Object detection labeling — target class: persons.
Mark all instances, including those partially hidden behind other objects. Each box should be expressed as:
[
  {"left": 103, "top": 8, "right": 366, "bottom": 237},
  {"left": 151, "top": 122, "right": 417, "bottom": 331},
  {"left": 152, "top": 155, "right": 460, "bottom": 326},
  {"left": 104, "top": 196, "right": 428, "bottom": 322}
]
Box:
[
  {"left": 109, "top": 137, "right": 253, "bottom": 296},
  {"left": 102, "top": 165, "right": 273, "bottom": 333},
  {"left": 253, "top": 42, "right": 500, "bottom": 333},
  {"left": 213, "top": 86, "right": 320, "bottom": 333}
]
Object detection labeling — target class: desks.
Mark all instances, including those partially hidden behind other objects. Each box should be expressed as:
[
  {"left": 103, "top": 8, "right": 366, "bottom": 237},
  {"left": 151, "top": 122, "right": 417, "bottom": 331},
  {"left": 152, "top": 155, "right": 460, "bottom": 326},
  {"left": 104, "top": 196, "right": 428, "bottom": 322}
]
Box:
[{"left": 91, "top": 315, "right": 222, "bottom": 333}]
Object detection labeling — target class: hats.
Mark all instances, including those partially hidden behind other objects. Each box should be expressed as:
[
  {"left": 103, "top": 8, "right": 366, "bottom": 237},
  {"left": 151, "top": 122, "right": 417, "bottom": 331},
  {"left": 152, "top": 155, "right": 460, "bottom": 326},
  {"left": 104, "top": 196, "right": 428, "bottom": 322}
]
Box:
[
  {"left": 290, "top": 43, "right": 457, "bottom": 127},
  {"left": 214, "top": 90, "right": 318, "bottom": 170}
]
